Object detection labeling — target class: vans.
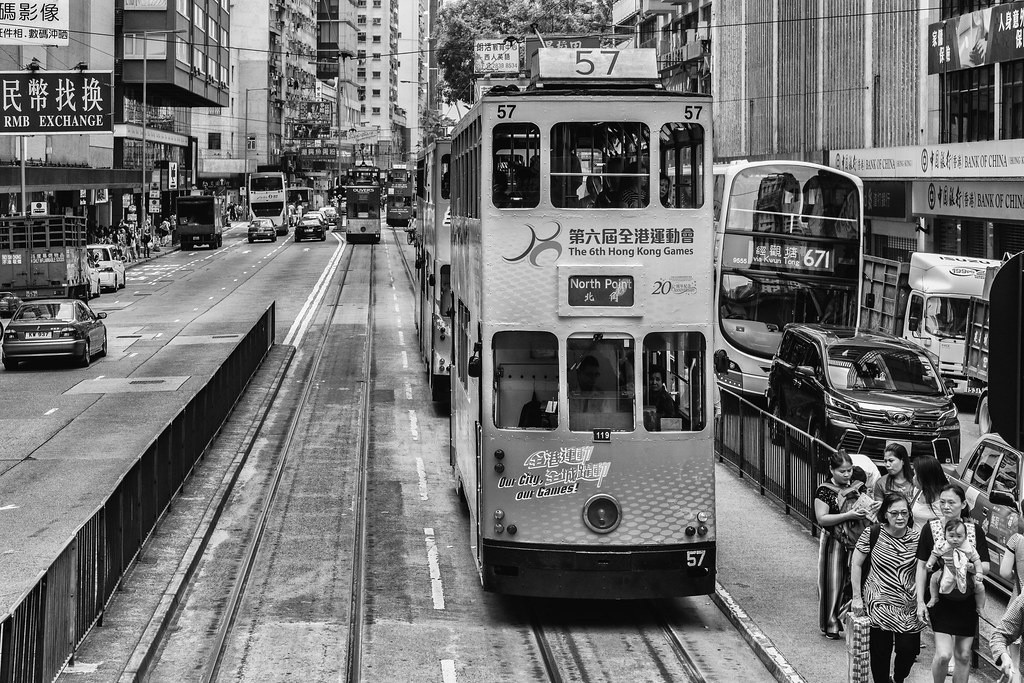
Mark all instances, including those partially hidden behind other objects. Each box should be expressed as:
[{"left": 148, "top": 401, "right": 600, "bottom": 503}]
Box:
[
  {"left": 88, "top": 244, "right": 126, "bottom": 290},
  {"left": 766, "top": 323, "right": 961, "bottom": 474}
]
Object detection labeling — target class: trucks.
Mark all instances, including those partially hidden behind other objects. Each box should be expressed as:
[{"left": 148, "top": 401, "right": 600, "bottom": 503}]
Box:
[
  {"left": 0, "top": 216, "right": 91, "bottom": 305},
  {"left": 860, "top": 249, "right": 1018, "bottom": 439},
  {"left": 175, "top": 196, "right": 223, "bottom": 249}
]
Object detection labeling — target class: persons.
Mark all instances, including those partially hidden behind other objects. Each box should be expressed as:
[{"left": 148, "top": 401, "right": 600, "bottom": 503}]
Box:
[
  {"left": 926, "top": 519, "right": 984, "bottom": 594},
  {"left": 814, "top": 451, "right": 869, "bottom": 639},
  {"left": 871, "top": 443, "right": 916, "bottom": 510},
  {"left": 569, "top": 355, "right": 612, "bottom": 413},
  {"left": 989, "top": 593, "right": 1024, "bottom": 678},
  {"left": 851, "top": 494, "right": 928, "bottom": 683},
  {"left": 644, "top": 363, "right": 674, "bottom": 417},
  {"left": 580, "top": 157, "right": 674, "bottom": 208},
  {"left": 516, "top": 136, "right": 583, "bottom": 191},
  {"left": 228, "top": 202, "right": 242, "bottom": 221},
  {"left": 91, "top": 220, "right": 152, "bottom": 258},
  {"left": 721, "top": 291, "right": 746, "bottom": 319},
  {"left": 160, "top": 214, "right": 176, "bottom": 246},
  {"left": 999, "top": 532, "right": 1024, "bottom": 598},
  {"left": 713, "top": 373, "right": 721, "bottom": 421},
  {"left": 914, "top": 483, "right": 992, "bottom": 683},
  {"left": 290, "top": 202, "right": 304, "bottom": 216},
  {"left": 909, "top": 454, "right": 955, "bottom": 675}
]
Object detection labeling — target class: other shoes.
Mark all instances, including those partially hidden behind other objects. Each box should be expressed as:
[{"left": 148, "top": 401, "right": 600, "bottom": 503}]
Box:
[
  {"left": 822, "top": 630, "right": 840, "bottom": 640},
  {"left": 947, "top": 664, "right": 955, "bottom": 676},
  {"left": 920, "top": 640, "right": 927, "bottom": 648}
]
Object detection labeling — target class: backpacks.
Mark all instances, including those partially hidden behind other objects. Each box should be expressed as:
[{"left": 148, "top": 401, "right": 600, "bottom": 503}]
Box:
[{"left": 816, "top": 481, "right": 879, "bottom": 548}]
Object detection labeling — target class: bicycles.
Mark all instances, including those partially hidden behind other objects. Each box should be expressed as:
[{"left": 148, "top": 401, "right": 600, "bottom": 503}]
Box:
[{"left": 404, "top": 227, "right": 417, "bottom": 245}]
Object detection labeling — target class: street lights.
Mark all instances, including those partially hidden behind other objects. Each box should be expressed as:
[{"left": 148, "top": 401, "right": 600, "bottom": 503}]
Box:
[
  {"left": 244, "top": 86, "right": 275, "bottom": 219},
  {"left": 400, "top": 80, "right": 430, "bottom": 148},
  {"left": 141, "top": 29, "right": 190, "bottom": 232}
]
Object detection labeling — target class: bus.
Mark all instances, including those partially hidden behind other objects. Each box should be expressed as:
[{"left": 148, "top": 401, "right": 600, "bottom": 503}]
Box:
[
  {"left": 666, "top": 159, "right": 875, "bottom": 395},
  {"left": 287, "top": 187, "right": 325, "bottom": 210},
  {"left": 387, "top": 168, "right": 413, "bottom": 224},
  {"left": 248, "top": 172, "right": 289, "bottom": 235},
  {"left": 413, "top": 48, "right": 720, "bottom": 600},
  {"left": 345, "top": 165, "right": 381, "bottom": 241}
]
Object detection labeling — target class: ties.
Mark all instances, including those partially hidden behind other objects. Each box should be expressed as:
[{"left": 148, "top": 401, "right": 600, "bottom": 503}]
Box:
[{"left": 582, "top": 399, "right": 589, "bottom": 413}]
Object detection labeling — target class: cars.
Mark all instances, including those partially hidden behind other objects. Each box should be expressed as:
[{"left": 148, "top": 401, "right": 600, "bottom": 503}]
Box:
[
  {"left": 294, "top": 198, "right": 346, "bottom": 243},
  {"left": 2, "top": 300, "right": 109, "bottom": 370},
  {"left": 87, "top": 253, "right": 101, "bottom": 297},
  {"left": 941, "top": 431, "right": 1023, "bottom": 587},
  {"left": 248, "top": 219, "right": 277, "bottom": 243}
]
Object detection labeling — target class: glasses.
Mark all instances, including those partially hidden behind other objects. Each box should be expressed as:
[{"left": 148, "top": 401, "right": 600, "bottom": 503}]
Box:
[
  {"left": 582, "top": 372, "right": 600, "bottom": 378},
  {"left": 887, "top": 510, "right": 910, "bottom": 517}
]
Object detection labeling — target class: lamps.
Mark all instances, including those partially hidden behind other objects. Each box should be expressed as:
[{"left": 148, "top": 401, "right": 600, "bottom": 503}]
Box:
[
  {"left": 916, "top": 222, "right": 929, "bottom": 234},
  {"left": 25, "top": 61, "right": 39, "bottom": 70},
  {"left": 69, "top": 61, "right": 88, "bottom": 70}
]
